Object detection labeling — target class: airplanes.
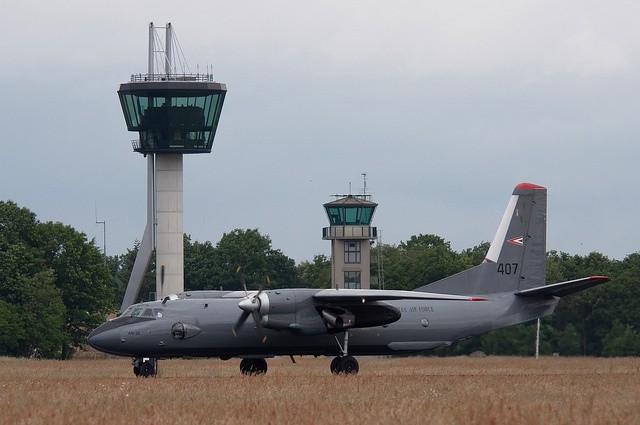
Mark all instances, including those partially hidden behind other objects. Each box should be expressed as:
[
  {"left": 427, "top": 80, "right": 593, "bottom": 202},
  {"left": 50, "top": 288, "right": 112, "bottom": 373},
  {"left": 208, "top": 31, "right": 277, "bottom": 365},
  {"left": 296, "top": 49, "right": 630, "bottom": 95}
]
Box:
[{"left": 87, "top": 182, "right": 612, "bottom": 378}]
